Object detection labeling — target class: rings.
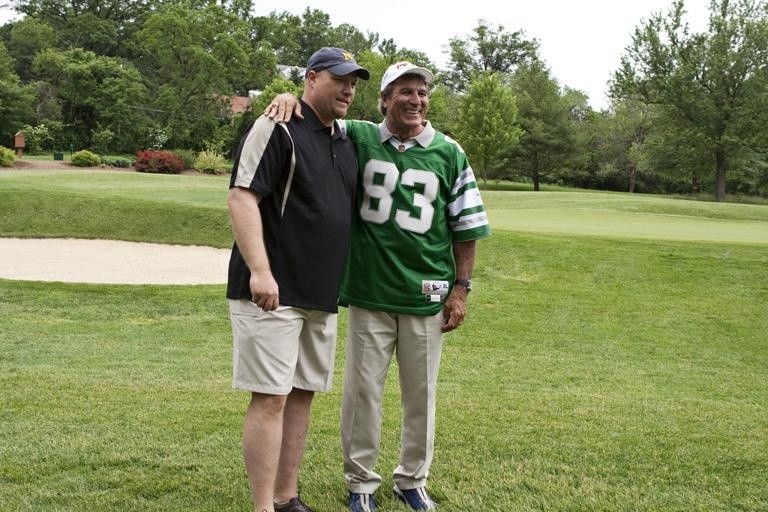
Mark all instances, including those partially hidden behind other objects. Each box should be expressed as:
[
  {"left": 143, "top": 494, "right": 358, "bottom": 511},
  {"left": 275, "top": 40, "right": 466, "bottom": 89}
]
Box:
[{"left": 271, "top": 103, "right": 278, "bottom": 107}]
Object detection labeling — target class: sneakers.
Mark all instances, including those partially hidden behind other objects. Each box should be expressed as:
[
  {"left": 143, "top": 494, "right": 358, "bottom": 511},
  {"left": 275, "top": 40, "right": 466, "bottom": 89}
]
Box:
[
  {"left": 347, "top": 489, "right": 376, "bottom": 512},
  {"left": 392, "top": 485, "right": 436, "bottom": 512}
]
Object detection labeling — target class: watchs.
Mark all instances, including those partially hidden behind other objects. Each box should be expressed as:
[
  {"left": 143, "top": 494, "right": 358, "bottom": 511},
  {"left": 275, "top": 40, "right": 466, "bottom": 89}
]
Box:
[{"left": 455, "top": 279, "right": 472, "bottom": 291}]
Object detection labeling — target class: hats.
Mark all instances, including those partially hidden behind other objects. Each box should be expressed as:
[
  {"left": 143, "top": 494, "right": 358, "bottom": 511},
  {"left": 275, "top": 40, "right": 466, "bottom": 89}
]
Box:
[
  {"left": 305, "top": 47, "right": 370, "bottom": 80},
  {"left": 380, "top": 61, "right": 435, "bottom": 93}
]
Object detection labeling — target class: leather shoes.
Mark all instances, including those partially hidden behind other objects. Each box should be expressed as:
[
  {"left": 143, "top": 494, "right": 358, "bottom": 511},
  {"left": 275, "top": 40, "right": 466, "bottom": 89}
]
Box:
[{"left": 274, "top": 498, "right": 315, "bottom": 512}]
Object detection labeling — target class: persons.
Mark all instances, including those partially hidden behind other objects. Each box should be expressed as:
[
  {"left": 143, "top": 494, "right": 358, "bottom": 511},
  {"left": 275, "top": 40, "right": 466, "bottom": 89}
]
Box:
[
  {"left": 226, "top": 46, "right": 359, "bottom": 512},
  {"left": 264, "top": 61, "right": 493, "bottom": 512}
]
